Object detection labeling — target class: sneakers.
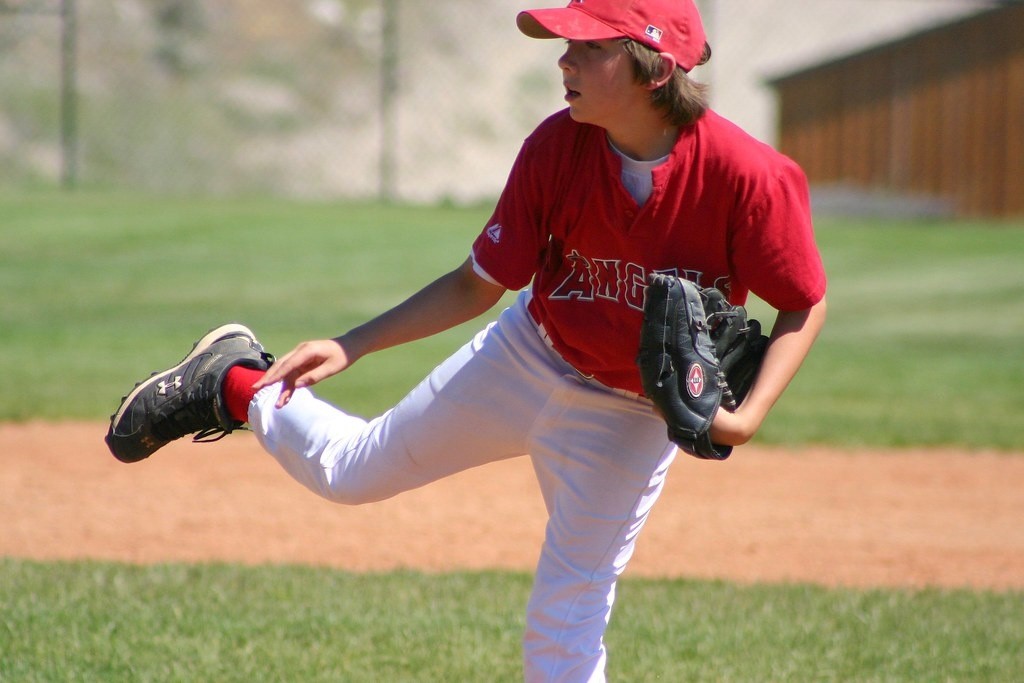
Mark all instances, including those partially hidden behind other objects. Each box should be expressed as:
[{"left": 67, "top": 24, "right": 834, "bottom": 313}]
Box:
[{"left": 104, "top": 321, "right": 280, "bottom": 464}]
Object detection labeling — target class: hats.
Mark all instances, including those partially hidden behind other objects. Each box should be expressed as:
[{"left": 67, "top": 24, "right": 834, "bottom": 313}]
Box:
[{"left": 516, "top": 0, "right": 706, "bottom": 74}]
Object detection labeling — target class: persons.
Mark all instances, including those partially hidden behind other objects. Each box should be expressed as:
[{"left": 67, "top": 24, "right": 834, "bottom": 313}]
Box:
[{"left": 105, "top": 0, "right": 826, "bottom": 683}]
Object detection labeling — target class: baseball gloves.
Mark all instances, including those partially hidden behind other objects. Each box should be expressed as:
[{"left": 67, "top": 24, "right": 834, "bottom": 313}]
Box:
[{"left": 637, "top": 273, "right": 769, "bottom": 461}]
